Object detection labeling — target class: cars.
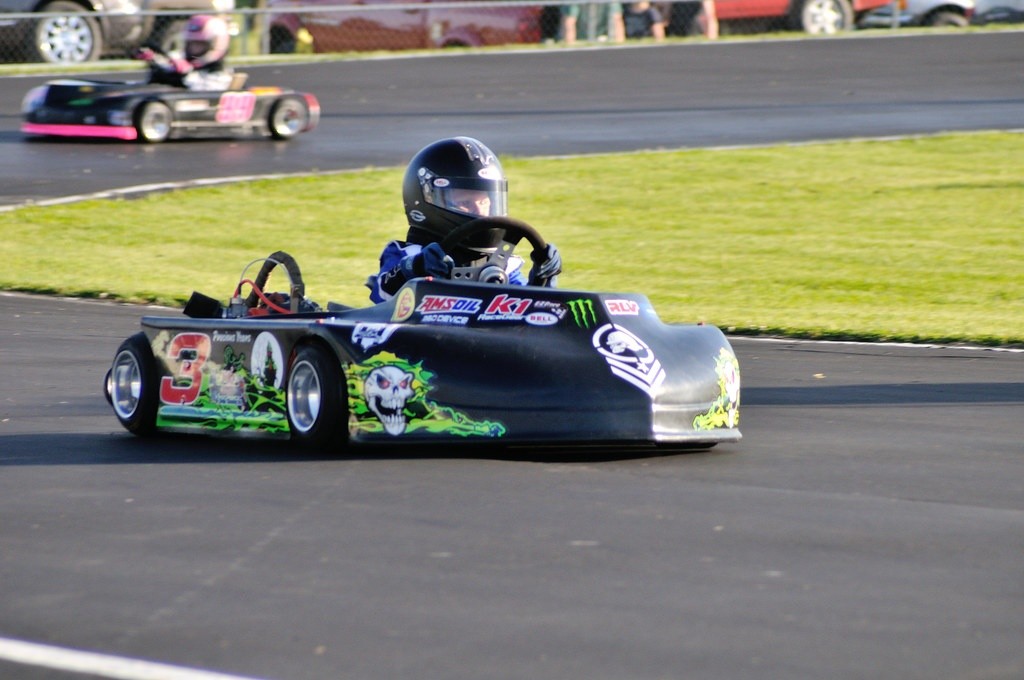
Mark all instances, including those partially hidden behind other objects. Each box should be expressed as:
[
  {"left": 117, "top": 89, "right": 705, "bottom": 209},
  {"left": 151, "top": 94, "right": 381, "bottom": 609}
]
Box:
[
  {"left": 858, "top": 0, "right": 1024, "bottom": 31},
  {"left": 0, "top": 1, "right": 238, "bottom": 63},
  {"left": 655, "top": 0, "right": 898, "bottom": 37},
  {"left": 259, "top": 0, "right": 564, "bottom": 56}
]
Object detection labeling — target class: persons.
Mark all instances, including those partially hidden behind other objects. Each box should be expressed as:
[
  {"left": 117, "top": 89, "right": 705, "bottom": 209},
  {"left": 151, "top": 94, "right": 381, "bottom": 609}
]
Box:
[
  {"left": 369, "top": 137, "right": 559, "bottom": 303},
  {"left": 557, "top": 0, "right": 721, "bottom": 48},
  {"left": 138, "top": 14, "right": 233, "bottom": 91}
]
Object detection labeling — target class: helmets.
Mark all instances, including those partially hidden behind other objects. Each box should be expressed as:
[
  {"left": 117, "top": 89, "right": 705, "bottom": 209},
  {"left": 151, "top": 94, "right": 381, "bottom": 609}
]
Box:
[
  {"left": 183, "top": 15, "right": 230, "bottom": 68},
  {"left": 402, "top": 137, "right": 509, "bottom": 248}
]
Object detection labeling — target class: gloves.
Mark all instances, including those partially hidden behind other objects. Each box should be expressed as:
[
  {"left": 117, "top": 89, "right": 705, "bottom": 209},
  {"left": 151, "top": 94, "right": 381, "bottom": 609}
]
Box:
[
  {"left": 530, "top": 243, "right": 562, "bottom": 279},
  {"left": 421, "top": 242, "right": 454, "bottom": 279}
]
